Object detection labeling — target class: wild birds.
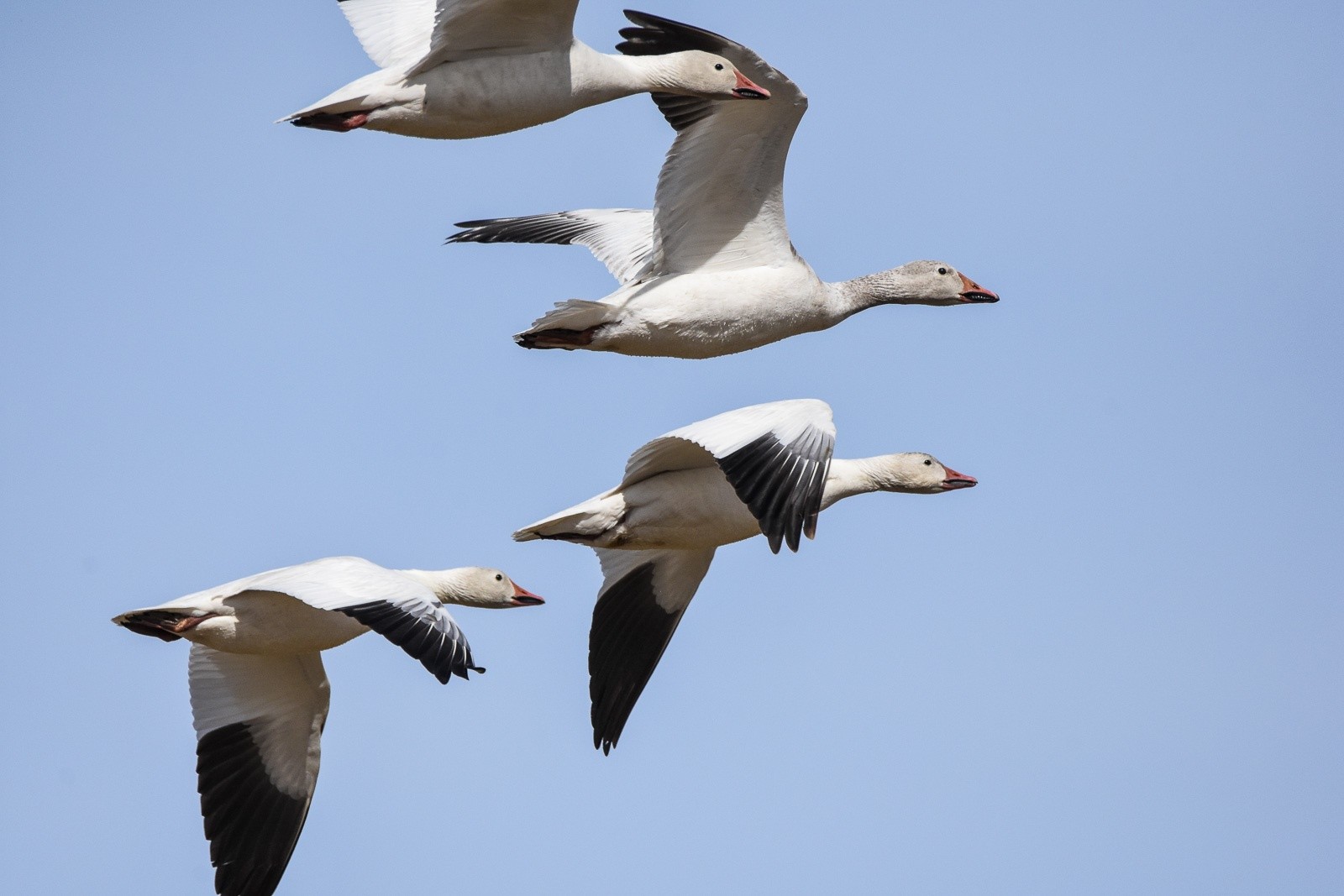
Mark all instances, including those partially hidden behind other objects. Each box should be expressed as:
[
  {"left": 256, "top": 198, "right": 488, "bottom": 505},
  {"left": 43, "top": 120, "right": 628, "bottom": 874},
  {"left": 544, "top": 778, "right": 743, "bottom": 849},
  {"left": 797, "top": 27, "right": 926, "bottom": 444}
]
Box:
[
  {"left": 509, "top": 397, "right": 978, "bottom": 757},
  {"left": 438, "top": 8, "right": 1000, "bottom": 360},
  {"left": 275, "top": 0, "right": 772, "bottom": 140},
  {"left": 111, "top": 554, "right": 545, "bottom": 896}
]
